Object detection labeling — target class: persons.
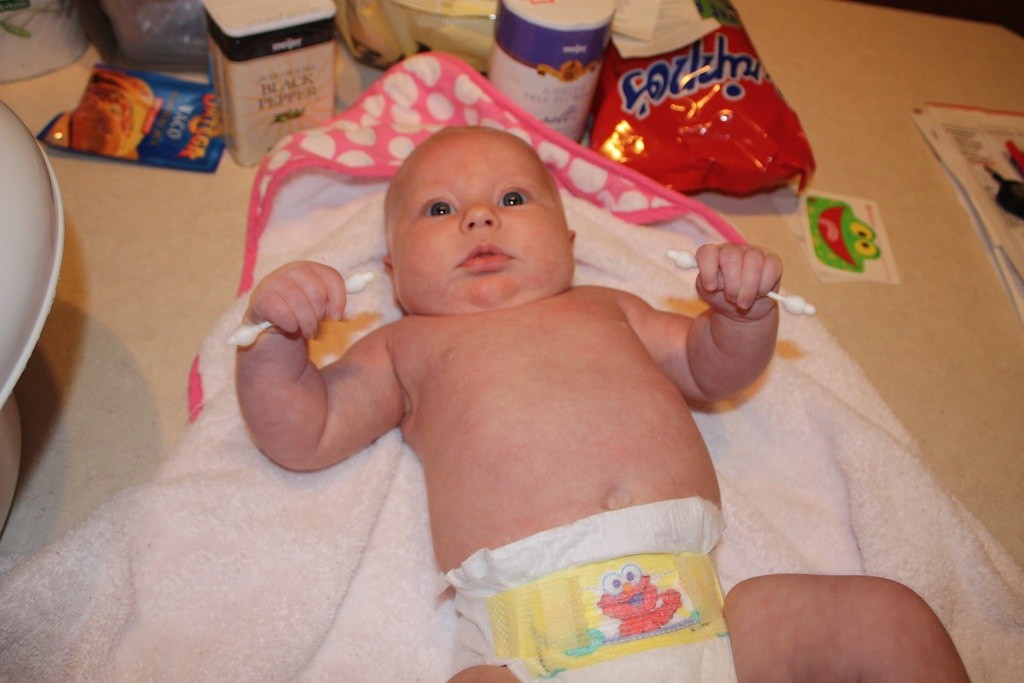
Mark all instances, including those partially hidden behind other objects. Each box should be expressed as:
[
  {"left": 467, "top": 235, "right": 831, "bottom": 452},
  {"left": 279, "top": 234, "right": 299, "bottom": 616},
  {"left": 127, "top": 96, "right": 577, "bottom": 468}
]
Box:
[{"left": 237, "top": 124, "right": 971, "bottom": 683}]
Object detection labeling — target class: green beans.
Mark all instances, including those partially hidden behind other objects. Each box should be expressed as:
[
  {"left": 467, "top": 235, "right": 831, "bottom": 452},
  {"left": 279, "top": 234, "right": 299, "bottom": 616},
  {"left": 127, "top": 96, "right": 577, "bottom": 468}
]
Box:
[{"left": 0, "top": 0, "right": 33, "bottom": 37}]
